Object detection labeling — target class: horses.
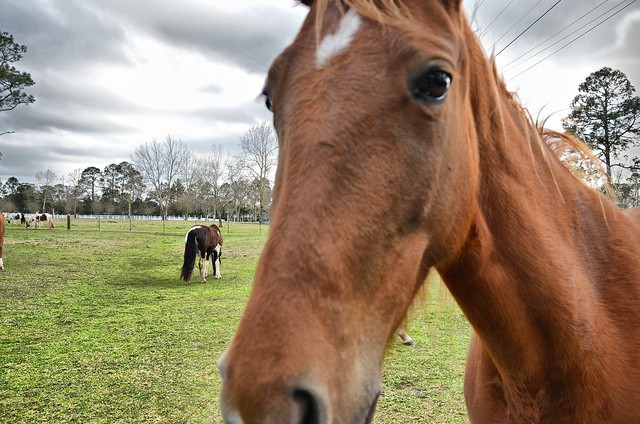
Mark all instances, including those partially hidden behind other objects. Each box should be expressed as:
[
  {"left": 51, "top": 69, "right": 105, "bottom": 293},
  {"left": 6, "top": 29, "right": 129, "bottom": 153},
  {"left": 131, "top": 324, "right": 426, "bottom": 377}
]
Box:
[
  {"left": 179, "top": 222, "right": 226, "bottom": 285},
  {"left": 0, "top": 210, "right": 55, "bottom": 272},
  {"left": 215, "top": 0, "right": 639, "bottom": 423}
]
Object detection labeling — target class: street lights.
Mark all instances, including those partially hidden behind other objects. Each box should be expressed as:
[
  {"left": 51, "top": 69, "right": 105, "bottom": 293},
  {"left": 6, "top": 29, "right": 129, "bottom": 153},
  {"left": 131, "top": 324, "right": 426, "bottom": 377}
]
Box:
[{"left": 0, "top": 130, "right": 15, "bottom": 138}]
